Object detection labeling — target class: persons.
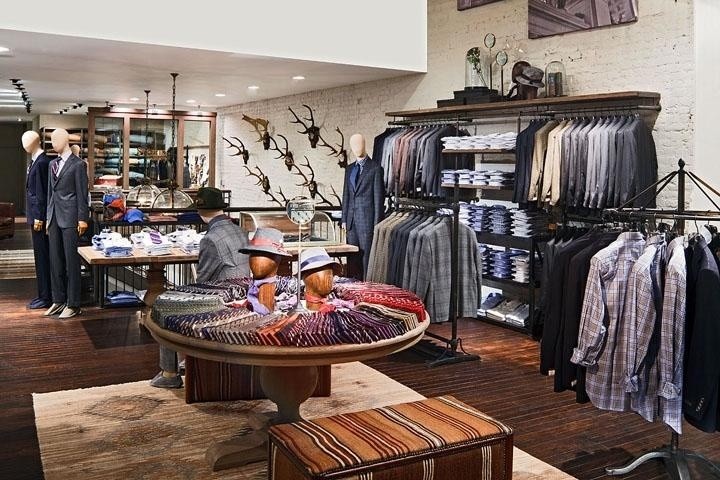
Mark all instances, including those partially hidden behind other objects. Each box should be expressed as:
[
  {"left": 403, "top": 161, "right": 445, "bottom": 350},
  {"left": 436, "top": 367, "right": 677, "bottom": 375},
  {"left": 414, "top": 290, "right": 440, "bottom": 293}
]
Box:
[
  {"left": 150, "top": 145, "right": 192, "bottom": 189},
  {"left": 70, "top": 143, "right": 80, "bottom": 156},
  {"left": 45, "top": 128, "right": 90, "bottom": 318},
  {"left": 294, "top": 249, "right": 343, "bottom": 310},
  {"left": 152, "top": 188, "right": 249, "bottom": 390},
  {"left": 240, "top": 226, "right": 292, "bottom": 308},
  {"left": 342, "top": 133, "right": 384, "bottom": 282},
  {"left": 20, "top": 131, "right": 49, "bottom": 308}
]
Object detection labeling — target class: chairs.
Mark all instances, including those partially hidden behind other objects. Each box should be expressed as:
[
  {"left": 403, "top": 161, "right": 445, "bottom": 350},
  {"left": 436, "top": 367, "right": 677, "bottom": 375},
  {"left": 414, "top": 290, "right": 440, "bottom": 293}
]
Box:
[{"left": 0, "top": 202, "right": 16, "bottom": 238}]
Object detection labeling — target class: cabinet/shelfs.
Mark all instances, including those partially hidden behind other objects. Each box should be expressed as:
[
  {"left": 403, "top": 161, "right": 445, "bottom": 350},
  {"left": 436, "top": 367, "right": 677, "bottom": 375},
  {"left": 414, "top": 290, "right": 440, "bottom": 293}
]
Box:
[
  {"left": 469, "top": 232, "right": 543, "bottom": 340},
  {"left": 441, "top": 149, "right": 517, "bottom": 191},
  {"left": 41, "top": 128, "right": 166, "bottom": 187}
]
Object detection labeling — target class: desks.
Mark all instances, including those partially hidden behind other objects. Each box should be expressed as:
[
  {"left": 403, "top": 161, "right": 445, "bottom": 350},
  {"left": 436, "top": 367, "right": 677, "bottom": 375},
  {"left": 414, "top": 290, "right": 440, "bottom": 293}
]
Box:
[
  {"left": 78, "top": 241, "right": 359, "bottom": 328},
  {"left": 145, "top": 276, "right": 430, "bottom": 472}
]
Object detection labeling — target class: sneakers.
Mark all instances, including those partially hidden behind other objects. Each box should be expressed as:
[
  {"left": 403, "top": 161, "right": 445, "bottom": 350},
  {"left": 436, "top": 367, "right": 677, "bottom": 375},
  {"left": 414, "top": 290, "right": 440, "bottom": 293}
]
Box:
[
  {"left": 59, "top": 307, "right": 82, "bottom": 318},
  {"left": 44, "top": 303, "right": 65, "bottom": 315},
  {"left": 28, "top": 298, "right": 48, "bottom": 309},
  {"left": 150, "top": 371, "right": 183, "bottom": 388}
]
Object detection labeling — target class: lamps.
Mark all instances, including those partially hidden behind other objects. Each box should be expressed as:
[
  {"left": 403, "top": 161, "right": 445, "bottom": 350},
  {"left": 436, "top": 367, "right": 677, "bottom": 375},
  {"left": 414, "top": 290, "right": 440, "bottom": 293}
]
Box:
[
  {"left": 152, "top": 72, "right": 194, "bottom": 208},
  {"left": 127, "top": 90, "right": 165, "bottom": 205}
]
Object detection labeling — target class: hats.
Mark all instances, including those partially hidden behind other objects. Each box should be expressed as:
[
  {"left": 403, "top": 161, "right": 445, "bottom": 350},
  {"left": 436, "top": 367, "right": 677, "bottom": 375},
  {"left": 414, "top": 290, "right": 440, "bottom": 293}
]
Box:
[
  {"left": 238, "top": 227, "right": 291, "bottom": 257},
  {"left": 292, "top": 247, "right": 342, "bottom": 279},
  {"left": 124, "top": 209, "right": 143, "bottom": 223},
  {"left": 515, "top": 67, "right": 544, "bottom": 88},
  {"left": 186, "top": 187, "right": 229, "bottom": 209},
  {"left": 107, "top": 199, "right": 125, "bottom": 213}
]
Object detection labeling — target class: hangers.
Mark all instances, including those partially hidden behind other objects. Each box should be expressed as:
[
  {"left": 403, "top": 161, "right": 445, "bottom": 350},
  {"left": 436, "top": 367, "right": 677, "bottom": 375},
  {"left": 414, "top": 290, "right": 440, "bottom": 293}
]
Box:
[
  {"left": 388, "top": 117, "right": 469, "bottom": 133},
  {"left": 395, "top": 200, "right": 451, "bottom": 224},
  {"left": 530, "top": 105, "right": 639, "bottom": 118},
  {"left": 554, "top": 212, "right": 718, "bottom": 237}
]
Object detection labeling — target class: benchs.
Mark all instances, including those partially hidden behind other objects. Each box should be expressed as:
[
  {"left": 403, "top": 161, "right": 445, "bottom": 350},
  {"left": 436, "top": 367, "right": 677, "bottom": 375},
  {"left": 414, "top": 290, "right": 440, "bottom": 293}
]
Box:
[
  {"left": 265, "top": 395, "right": 517, "bottom": 480},
  {"left": 186, "top": 350, "right": 331, "bottom": 404}
]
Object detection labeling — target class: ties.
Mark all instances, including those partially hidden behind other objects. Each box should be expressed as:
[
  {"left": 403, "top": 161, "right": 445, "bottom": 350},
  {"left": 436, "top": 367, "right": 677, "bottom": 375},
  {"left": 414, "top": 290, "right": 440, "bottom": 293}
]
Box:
[
  {"left": 26, "top": 160, "right": 34, "bottom": 189},
  {"left": 150, "top": 229, "right": 162, "bottom": 245},
  {"left": 52, "top": 158, "right": 62, "bottom": 180},
  {"left": 350, "top": 163, "right": 360, "bottom": 191}
]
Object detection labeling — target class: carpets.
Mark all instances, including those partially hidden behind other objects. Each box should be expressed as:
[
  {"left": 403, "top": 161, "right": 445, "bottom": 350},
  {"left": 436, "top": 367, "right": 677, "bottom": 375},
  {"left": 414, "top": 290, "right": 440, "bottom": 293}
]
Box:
[{"left": 31, "top": 362, "right": 581, "bottom": 480}]
